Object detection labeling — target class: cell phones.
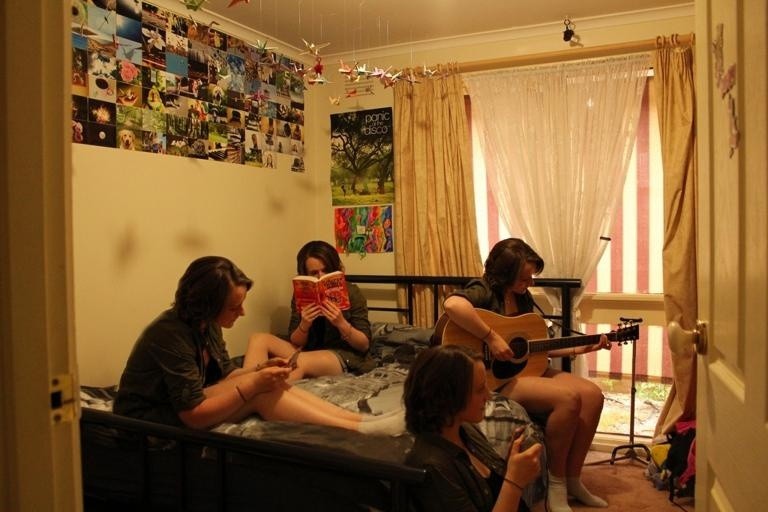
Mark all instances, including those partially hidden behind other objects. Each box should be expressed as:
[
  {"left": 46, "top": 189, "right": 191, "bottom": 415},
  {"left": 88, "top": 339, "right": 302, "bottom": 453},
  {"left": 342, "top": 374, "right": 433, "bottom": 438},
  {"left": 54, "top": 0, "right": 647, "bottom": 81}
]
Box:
[{"left": 285, "top": 347, "right": 302, "bottom": 367}]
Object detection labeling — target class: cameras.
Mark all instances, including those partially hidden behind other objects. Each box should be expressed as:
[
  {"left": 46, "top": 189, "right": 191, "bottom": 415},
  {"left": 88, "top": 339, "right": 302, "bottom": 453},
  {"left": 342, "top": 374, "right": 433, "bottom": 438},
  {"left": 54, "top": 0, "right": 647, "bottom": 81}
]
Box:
[{"left": 521, "top": 423, "right": 544, "bottom": 452}]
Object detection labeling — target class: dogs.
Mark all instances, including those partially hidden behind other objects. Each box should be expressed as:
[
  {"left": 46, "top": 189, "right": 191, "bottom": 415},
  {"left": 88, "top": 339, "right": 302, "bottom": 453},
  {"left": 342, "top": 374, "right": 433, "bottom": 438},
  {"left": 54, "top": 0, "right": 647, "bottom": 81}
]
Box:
[
  {"left": 118, "top": 129, "right": 136, "bottom": 151},
  {"left": 210, "top": 87, "right": 224, "bottom": 104}
]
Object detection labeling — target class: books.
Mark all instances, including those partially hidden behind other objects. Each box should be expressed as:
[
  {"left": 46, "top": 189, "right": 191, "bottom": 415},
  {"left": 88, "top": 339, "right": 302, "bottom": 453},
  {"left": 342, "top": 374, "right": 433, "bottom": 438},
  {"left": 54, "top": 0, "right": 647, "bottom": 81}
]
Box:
[{"left": 292, "top": 269, "right": 352, "bottom": 320}]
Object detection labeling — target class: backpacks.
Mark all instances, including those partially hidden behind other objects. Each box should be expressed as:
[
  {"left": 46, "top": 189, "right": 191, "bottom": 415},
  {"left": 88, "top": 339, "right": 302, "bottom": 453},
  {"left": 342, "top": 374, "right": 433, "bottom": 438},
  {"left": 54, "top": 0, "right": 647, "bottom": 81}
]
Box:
[{"left": 659, "top": 419, "right": 697, "bottom": 495}]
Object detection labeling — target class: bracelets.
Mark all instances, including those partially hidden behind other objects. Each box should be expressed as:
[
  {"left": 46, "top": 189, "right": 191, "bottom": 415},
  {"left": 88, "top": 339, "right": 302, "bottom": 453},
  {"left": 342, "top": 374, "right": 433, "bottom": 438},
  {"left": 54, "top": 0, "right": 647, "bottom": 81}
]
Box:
[
  {"left": 480, "top": 327, "right": 492, "bottom": 340},
  {"left": 297, "top": 324, "right": 310, "bottom": 333},
  {"left": 572, "top": 346, "right": 576, "bottom": 357},
  {"left": 340, "top": 324, "right": 353, "bottom": 342},
  {"left": 234, "top": 384, "right": 247, "bottom": 404},
  {"left": 501, "top": 476, "right": 527, "bottom": 494}
]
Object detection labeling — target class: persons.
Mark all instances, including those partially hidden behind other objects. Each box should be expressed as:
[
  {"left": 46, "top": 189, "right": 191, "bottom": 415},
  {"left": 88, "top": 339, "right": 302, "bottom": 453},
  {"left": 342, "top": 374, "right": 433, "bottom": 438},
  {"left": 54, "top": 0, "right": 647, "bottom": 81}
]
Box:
[
  {"left": 241, "top": 240, "right": 374, "bottom": 379},
  {"left": 441, "top": 237, "right": 615, "bottom": 511},
  {"left": 114, "top": 255, "right": 409, "bottom": 444},
  {"left": 385, "top": 343, "right": 546, "bottom": 512}
]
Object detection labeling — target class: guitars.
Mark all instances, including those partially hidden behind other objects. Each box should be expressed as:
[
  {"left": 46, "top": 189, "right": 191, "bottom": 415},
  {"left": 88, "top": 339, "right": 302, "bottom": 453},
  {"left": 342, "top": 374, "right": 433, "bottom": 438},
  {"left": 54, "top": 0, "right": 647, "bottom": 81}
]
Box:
[{"left": 435, "top": 307, "right": 640, "bottom": 393}]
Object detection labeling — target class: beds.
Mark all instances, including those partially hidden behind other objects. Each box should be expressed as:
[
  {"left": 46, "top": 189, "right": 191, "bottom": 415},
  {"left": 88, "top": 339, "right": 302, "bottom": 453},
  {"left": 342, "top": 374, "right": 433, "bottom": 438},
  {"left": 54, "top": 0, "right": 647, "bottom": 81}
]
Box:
[{"left": 82, "top": 274, "right": 582, "bottom": 511}]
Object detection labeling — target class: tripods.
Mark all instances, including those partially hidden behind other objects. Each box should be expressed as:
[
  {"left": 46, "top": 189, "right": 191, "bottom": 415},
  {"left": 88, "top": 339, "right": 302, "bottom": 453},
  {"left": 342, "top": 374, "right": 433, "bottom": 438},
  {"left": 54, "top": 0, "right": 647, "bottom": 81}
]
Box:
[{"left": 585, "top": 322, "right": 652, "bottom": 466}]
